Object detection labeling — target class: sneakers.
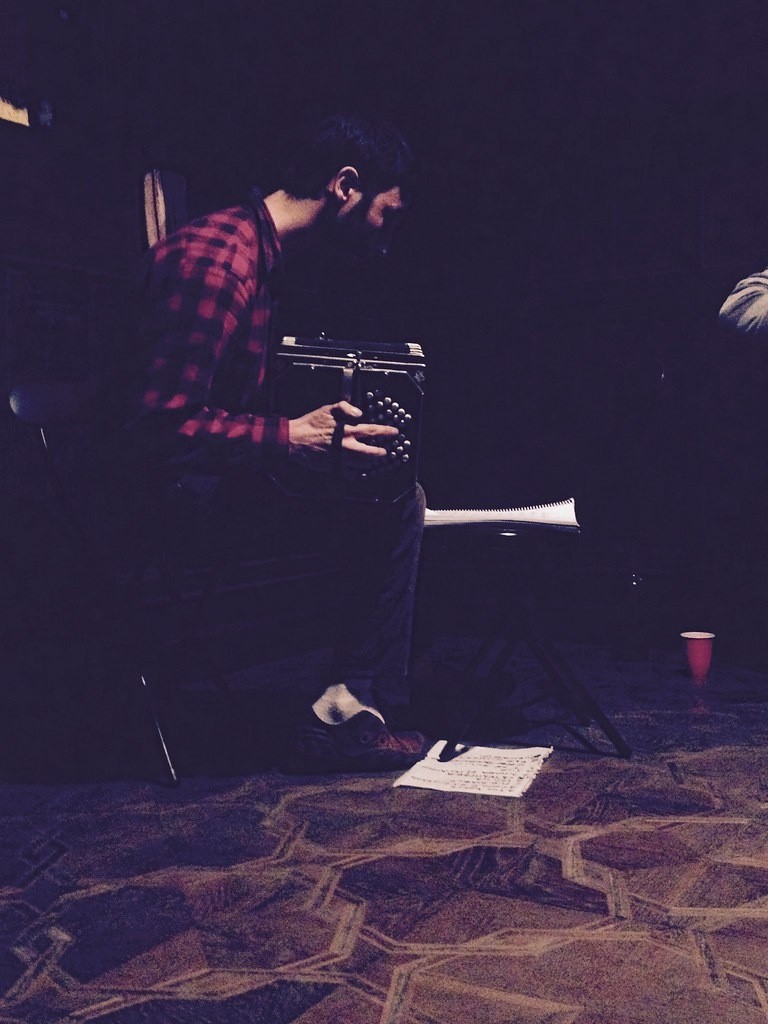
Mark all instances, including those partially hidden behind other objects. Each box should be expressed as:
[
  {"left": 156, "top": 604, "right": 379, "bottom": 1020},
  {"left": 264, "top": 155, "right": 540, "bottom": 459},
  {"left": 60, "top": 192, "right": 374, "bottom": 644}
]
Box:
[{"left": 276, "top": 720, "right": 429, "bottom": 776}]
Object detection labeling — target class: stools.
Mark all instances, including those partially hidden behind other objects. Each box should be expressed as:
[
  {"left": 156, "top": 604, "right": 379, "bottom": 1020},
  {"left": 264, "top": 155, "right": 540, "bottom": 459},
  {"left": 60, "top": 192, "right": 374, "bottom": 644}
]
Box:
[{"left": 439, "top": 529, "right": 634, "bottom": 761}]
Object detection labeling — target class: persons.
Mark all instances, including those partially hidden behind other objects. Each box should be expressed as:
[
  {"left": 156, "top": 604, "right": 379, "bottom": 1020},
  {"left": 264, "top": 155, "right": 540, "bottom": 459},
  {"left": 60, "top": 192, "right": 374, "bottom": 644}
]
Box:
[{"left": 142, "top": 121, "right": 430, "bottom": 770}]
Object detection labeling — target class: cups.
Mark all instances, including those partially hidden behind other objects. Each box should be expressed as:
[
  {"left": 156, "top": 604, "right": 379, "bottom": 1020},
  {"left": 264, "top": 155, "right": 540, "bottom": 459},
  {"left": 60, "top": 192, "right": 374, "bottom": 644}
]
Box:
[{"left": 680, "top": 631, "right": 715, "bottom": 678}]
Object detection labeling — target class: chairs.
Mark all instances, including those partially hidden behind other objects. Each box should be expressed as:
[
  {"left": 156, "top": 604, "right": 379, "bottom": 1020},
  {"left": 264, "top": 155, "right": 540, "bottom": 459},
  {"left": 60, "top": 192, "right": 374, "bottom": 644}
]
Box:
[{"left": 12, "top": 376, "right": 255, "bottom": 785}]
